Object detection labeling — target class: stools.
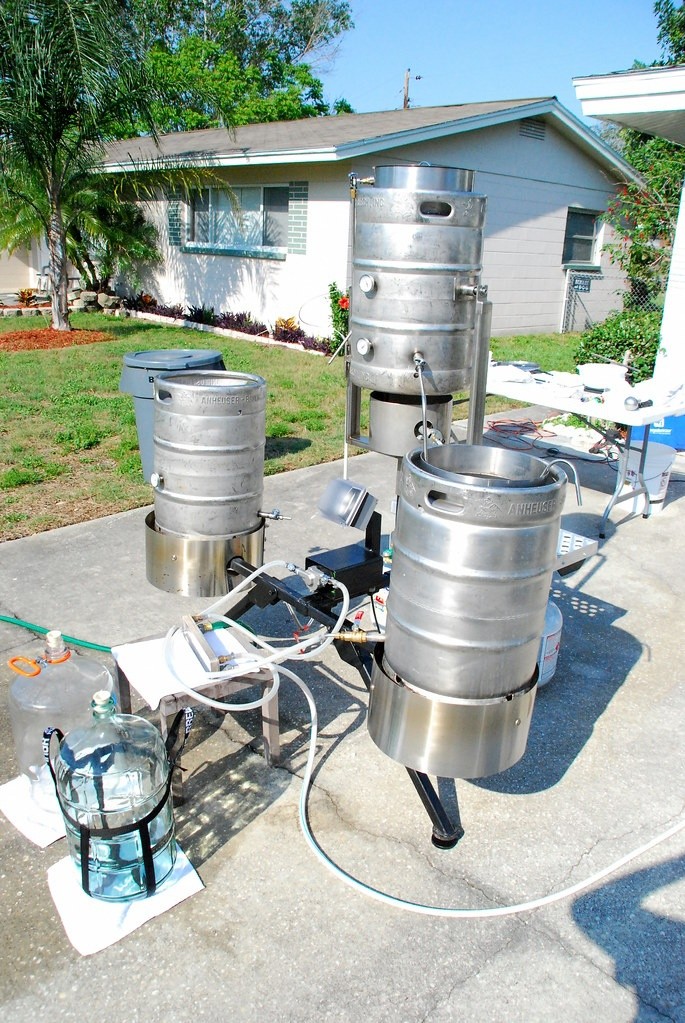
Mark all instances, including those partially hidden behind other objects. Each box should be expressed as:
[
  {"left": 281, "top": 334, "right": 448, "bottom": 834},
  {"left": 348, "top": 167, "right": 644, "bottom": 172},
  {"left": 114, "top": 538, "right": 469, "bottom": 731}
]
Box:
[{"left": 112, "top": 620, "right": 281, "bottom": 809}]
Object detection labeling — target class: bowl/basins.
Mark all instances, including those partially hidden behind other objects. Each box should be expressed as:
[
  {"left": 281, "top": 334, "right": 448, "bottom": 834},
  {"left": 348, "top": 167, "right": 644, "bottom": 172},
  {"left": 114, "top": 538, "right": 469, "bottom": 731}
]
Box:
[{"left": 577, "top": 362, "right": 628, "bottom": 388}]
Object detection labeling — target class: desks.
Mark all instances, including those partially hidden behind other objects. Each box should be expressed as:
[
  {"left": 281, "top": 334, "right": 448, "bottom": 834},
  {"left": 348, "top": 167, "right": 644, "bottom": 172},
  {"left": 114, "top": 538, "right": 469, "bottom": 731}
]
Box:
[{"left": 482, "top": 365, "right": 685, "bottom": 539}]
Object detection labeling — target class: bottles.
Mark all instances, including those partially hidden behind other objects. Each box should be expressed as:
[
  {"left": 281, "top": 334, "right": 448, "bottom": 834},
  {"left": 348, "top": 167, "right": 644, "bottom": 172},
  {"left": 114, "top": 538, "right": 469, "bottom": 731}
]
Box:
[
  {"left": 53, "top": 690, "right": 177, "bottom": 903},
  {"left": 8, "top": 631, "right": 115, "bottom": 815}
]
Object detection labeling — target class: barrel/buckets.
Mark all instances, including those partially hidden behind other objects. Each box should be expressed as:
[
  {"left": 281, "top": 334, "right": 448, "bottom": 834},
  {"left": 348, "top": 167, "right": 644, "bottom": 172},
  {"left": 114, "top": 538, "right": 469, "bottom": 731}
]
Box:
[
  {"left": 118, "top": 349, "right": 227, "bottom": 483},
  {"left": 616, "top": 440, "right": 677, "bottom": 513}
]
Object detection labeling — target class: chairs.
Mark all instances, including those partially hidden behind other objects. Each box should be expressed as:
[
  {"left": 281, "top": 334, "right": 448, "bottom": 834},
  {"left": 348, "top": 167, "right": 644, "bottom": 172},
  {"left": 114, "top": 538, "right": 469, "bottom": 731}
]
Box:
[{"left": 35, "top": 260, "right": 79, "bottom": 293}]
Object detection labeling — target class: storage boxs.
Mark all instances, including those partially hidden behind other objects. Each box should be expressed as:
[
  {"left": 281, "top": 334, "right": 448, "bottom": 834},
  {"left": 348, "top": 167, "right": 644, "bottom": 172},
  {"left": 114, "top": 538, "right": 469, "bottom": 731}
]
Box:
[{"left": 630, "top": 414, "right": 685, "bottom": 452}]
